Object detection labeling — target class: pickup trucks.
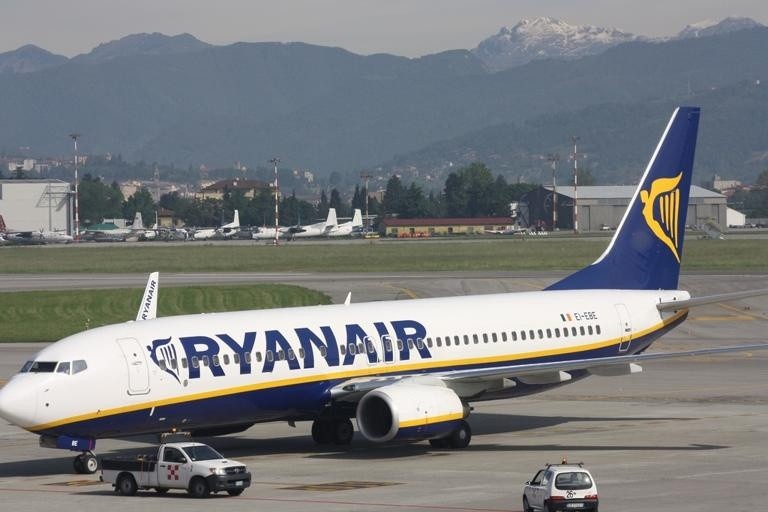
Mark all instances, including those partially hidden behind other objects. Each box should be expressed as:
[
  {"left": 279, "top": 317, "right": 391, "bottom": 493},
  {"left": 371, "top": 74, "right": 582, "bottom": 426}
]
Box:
[{"left": 99, "top": 428, "right": 251, "bottom": 498}]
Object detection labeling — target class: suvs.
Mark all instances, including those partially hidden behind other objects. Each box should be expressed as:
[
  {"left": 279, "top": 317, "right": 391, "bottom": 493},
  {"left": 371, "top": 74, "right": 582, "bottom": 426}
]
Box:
[{"left": 521, "top": 456, "right": 599, "bottom": 512}]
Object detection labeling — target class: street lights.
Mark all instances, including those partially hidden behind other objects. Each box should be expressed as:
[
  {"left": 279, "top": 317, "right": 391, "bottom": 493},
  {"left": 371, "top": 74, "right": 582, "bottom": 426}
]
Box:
[
  {"left": 361, "top": 175, "right": 373, "bottom": 236},
  {"left": 548, "top": 156, "right": 560, "bottom": 231},
  {"left": 569, "top": 134, "right": 588, "bottom": 233},
  {"left": 270, "top": 158, "right": 280, "bottom": 245},
  {"left": 69, "top": 133, "right": 82, "bottom": 242}
]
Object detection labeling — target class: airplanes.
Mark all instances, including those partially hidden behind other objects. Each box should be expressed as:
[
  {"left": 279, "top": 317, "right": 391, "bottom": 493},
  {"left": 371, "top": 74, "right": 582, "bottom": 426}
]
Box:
[{"left": 0, "top": 104, "right": 703, "bottom": 475}]
